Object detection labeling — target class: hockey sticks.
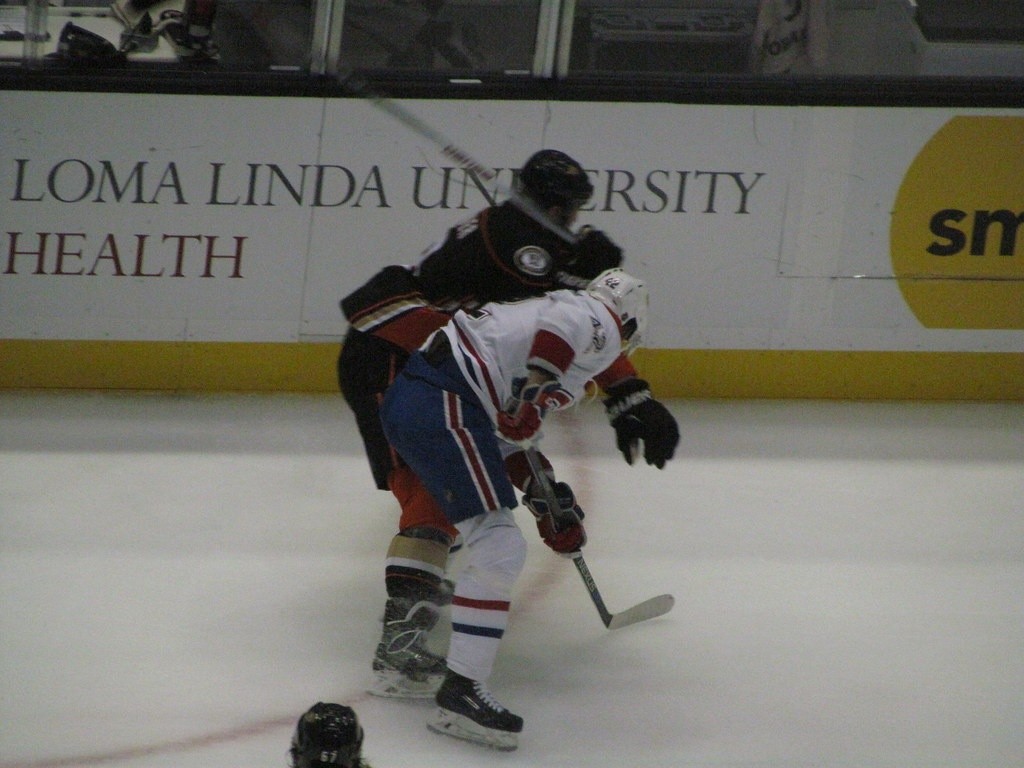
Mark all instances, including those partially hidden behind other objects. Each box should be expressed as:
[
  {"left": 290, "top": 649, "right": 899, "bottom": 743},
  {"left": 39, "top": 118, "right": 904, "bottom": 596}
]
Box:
[
  {"left": 504, "top": 399, "right": 676, "bottom": 632},
  {"left": 236, "top": 1, "right": 581, "bottom": 243}
]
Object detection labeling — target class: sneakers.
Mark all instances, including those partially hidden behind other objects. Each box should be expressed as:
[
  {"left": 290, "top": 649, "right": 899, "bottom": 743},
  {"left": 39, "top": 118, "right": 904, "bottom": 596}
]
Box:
[
  {"left": 427, "top": 669, "right": 523, "bottom": 751},
  {"left": 366, "top": 600, "right": 448, "bottom": 698}
]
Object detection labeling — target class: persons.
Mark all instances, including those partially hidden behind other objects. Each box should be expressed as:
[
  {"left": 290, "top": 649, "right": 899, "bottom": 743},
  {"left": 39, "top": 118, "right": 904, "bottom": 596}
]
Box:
[
  {"left": 290, "top": 701, "right": 364, "bottom": 768},
  {"left": 336, "top": 148, "right": 680, "bottom": 733}
]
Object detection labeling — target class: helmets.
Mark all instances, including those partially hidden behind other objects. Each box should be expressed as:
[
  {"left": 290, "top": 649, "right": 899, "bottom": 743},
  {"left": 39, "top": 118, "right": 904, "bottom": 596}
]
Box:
[
  {"left": 520, "top": 149, "right": 594, "bottom": 212},
  {"left": 298, "top": 701, "right": 364, "bottom": 768},
  {"left": 586, "top": 268, "right": 648, "bottom": 342}
]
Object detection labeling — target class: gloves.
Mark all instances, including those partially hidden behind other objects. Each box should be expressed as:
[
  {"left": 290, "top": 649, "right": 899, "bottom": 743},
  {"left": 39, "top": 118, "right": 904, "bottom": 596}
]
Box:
[
  {"left": 496, "top": 376, "right": 574, "bottom": 441},
  {"left": 601, "top": 379, "right": 678, "bottom": 469},
  {"left": 522, "top": 482, "right": 587, "bottom": 560}
]
